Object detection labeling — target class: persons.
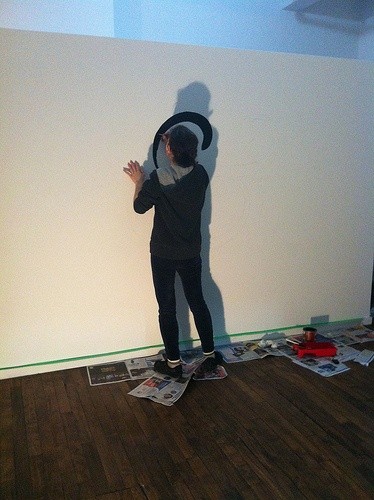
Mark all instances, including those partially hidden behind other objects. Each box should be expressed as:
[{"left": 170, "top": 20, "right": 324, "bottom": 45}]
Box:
[{"left": 122, "top": 123, "right": 224, "bottom": 382}]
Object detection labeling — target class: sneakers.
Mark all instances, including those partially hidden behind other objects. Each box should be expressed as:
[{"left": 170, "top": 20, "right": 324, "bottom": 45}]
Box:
[
  {"left": 180, "top": 352, "right": 217, "bottom": 370},
  {"left": 154, "top": 361, "right": 182, "bottom": 378}
]
